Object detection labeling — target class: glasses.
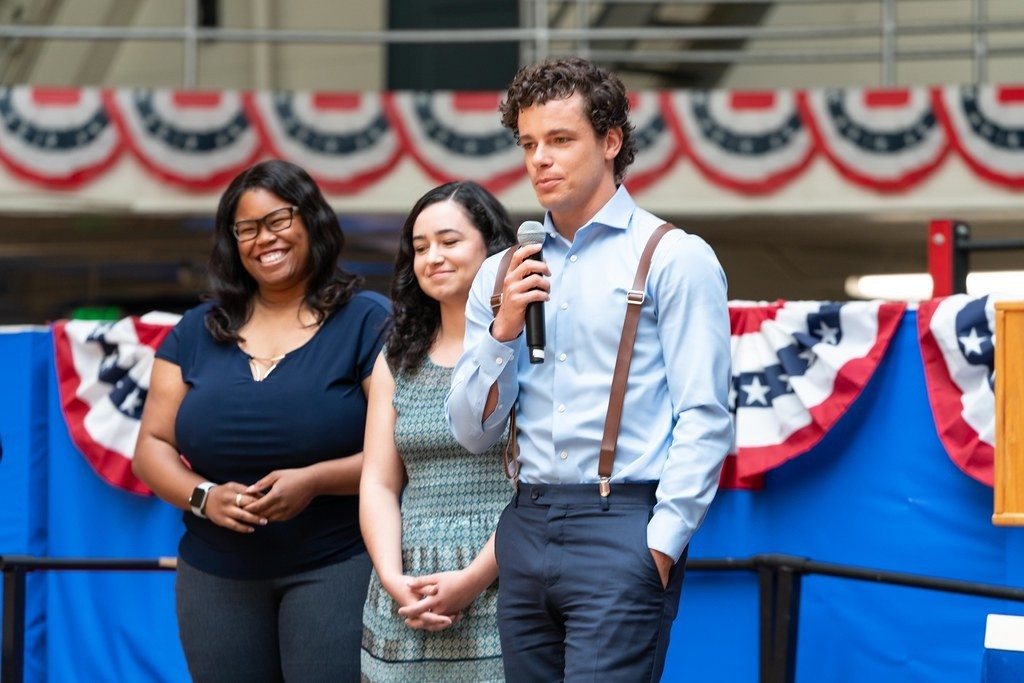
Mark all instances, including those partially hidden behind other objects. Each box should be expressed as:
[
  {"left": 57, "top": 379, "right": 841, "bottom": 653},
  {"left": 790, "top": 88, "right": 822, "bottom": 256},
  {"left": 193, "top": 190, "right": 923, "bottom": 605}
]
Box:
[{"left": 233, "top": 206, "right": 299, "bottom": 242}]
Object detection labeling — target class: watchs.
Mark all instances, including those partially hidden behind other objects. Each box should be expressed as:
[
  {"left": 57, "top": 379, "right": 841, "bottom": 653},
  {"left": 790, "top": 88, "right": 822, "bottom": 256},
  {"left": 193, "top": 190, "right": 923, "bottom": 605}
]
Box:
[{"left": 189, "top": 481, "right": 219, "bottom": 519}]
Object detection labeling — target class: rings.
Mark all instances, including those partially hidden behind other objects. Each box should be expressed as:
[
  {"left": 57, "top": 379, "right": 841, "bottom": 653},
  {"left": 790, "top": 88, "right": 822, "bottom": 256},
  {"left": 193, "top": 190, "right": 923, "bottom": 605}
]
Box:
[{"left": 235, "top": 493, "right": 244, "bottom": 508}]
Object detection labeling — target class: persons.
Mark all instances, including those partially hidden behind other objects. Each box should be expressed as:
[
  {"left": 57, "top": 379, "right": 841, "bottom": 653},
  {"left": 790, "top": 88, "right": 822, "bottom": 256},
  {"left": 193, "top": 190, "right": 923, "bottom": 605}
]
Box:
[
  {"left": 355, "top": 179, "right": 519, "bottom": 682},
  {"left": 130, "top": 157, "right": 393, "bottom": 683},
  {"left": 441, "top": 53, "right": 734, "bottom": 683}
]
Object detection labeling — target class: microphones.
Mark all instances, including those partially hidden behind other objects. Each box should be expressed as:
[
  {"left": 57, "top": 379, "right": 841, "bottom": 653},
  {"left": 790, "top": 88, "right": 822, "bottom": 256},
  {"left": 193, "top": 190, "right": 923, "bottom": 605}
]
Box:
[{"left": 517, "top": 221, "right": 547, "bottom": 363}]
